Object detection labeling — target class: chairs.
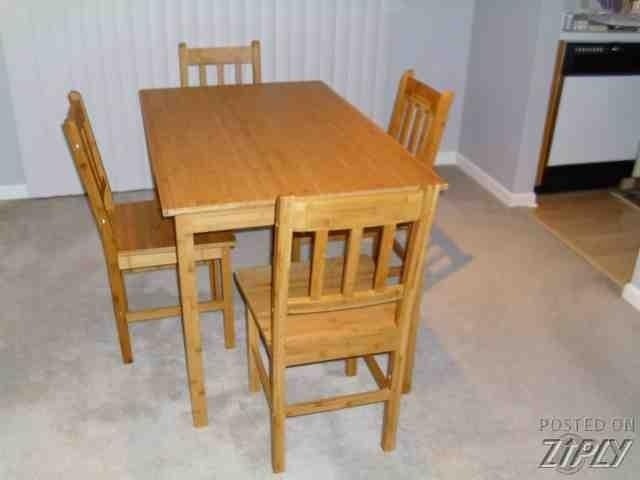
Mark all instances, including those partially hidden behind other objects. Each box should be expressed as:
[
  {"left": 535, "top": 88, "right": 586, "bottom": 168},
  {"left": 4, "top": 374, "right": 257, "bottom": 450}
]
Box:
[{"left": 60, "top": 40, "right": 456, "bottom": 473}]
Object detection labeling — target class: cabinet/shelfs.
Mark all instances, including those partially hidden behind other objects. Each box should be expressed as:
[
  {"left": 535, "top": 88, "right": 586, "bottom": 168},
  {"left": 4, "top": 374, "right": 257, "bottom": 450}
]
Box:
[{"left": 534, "top": 43, "right": 640, "bottom": 193}]
